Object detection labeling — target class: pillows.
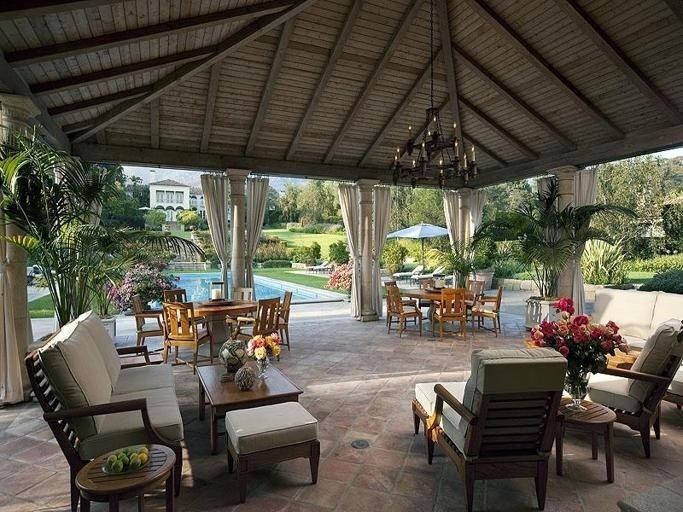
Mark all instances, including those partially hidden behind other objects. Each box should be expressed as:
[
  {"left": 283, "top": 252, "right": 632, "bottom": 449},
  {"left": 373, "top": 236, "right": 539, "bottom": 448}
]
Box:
[
  {"left": 459, "top": 349, "right": 564, "bottom": 437},
  {"left": 591, "top": 289, "right": 658, "bottom": 342},
  {"left": 649, "top": 291, "right": 682, "bottom": 337},
  {"left": 76, "top": 309, "right": 122, "bottom": 389},
  {"left": 39, "top": 320, "right": 114, "bottom": 440},
  {"left": 626, "top": 319, "right": 682, "bottom": 404}
]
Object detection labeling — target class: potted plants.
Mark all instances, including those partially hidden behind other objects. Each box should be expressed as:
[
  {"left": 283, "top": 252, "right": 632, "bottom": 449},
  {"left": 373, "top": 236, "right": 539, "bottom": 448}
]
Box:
[
  {"left": 433, "top": 236, "right": 497, "bottom": 297},
  {"left": 85, "top": 226, "right": 208, "bottom": 350},
  {"left": 469, "top": 169, "right": 640, "bottom": 330}
]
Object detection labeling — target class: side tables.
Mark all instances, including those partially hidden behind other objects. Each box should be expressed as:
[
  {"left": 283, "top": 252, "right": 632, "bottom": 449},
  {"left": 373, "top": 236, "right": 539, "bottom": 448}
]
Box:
[
  {"left": 556, "top": 398, "right": 616, "bottom": 483},
  {"left": 75, "top": 443, "right": 176, "bottom": 512}
]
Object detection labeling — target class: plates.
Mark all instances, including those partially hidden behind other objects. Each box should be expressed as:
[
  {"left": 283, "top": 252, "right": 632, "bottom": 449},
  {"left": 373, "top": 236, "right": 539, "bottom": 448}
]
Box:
[{"left": 102, "top": 455, "right": 151, "bottom": 475}]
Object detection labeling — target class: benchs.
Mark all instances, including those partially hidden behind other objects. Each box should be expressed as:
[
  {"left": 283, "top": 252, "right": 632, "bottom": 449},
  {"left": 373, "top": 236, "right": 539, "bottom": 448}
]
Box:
[{"left": 23, "top": 310, "right": 185, "bottom": 512}]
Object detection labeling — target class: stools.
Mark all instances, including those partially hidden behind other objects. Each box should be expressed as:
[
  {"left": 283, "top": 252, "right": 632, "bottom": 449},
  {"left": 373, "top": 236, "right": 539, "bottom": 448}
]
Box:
[{"left": 225, "top": 401, "right": 321, "bottom": 503}]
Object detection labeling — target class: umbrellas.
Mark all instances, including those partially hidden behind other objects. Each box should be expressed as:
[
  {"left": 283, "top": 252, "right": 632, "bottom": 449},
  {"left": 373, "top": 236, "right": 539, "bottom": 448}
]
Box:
[{"left": 385, "top": 220, "right": 449, "bottom": 275}]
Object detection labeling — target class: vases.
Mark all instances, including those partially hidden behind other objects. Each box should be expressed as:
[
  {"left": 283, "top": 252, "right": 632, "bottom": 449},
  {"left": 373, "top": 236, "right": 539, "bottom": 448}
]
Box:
[{"left": 562, "top": 376, "right": 587, "bottom": 414}]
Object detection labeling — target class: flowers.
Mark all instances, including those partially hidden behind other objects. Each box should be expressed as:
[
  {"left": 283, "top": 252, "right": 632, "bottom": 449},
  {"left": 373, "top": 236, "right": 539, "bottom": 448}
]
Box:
[{"left": 522, "top": 297, "right": 630, "bottom": 398}]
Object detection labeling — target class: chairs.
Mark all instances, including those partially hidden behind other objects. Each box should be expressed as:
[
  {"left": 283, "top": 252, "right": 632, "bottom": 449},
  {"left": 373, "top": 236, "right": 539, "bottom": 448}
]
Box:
[
  {"left": 380, "top": 265, "right": 502, "bottom": 341},
  {"left": 131, "top": 288, "right": 292, "bottom": 375},
  {"left": 617, "top": 357, "right": 683, "bottom": 410},
  {"left": 307, "top": 260, "right": 334, "bottom": 274},
  {"left": 413, "top": 346, "right": 568, "bottom": 511},
  {"left": 585, "top": 318, "right": 683, "bottom": 459}
]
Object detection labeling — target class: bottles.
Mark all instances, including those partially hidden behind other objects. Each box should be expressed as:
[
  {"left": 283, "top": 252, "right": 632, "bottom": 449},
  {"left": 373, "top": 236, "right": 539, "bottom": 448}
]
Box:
[
  {"left": 209, "top": 281, "right": 224, "bottom": 300},
  {"left": 432, "top": 274, "right": 444, "bottom": 289}
]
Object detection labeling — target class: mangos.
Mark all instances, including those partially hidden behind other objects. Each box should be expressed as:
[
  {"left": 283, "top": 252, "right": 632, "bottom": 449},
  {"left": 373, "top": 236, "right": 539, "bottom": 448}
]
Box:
[{"left": 106, "top": 447, "right": 149, "bottom": 473}]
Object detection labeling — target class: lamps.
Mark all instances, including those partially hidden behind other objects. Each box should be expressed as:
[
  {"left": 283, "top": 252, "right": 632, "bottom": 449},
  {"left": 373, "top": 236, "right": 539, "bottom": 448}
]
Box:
[{"left": 389, "top": 0, "right": 480, "bottom": 190}]
